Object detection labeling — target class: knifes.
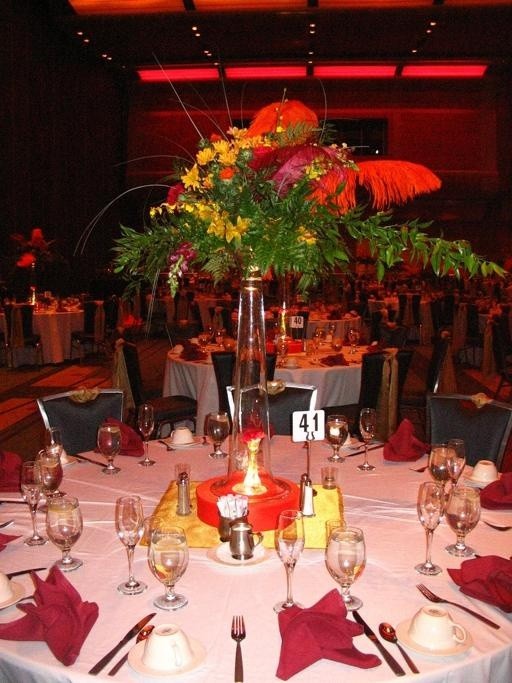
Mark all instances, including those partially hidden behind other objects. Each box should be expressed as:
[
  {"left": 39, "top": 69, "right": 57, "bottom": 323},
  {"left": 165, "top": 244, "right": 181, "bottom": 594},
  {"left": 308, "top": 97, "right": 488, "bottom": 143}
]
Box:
[
  {"left": 0, "top": 518, "right": 15, "bottom": 530},
  {"left": 350, "top": 609, "right": 406, "bottom": 676},
  {"left": 70, "top": 451, "right": 109, "bottom": 468},
  {"left": 89, "top": 612, "right": 158, "bottom": 675},
  {"left": 345, "top": 444, "right": 385, "bottom": 459},
  {"left": 5, "top": 566, "right": 48, "bottom": 580}
]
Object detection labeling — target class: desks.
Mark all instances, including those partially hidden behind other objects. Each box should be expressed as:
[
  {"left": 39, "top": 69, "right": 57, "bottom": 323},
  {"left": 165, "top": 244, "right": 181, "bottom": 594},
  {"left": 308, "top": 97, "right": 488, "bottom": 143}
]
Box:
[{"left": 2, "top": 431, "right": 510, "bottom": 678}]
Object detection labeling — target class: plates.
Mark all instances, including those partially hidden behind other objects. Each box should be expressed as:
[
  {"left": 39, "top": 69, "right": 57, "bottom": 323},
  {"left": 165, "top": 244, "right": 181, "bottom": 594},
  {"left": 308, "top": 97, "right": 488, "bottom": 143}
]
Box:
[
  {"left": 459, "top": 471, "right": 502, "bottom": 487},
  {"left": 128, "top": 636, "right": 207, "bottom": 678},
  {"left": 215, "top": 540, "right": 267, "bottom": 564},
  {"left": 0, "top": 490, "right": 25, "bottom": 505},
  {"left": 395, "top": 619, "right": 473, "bottom": 656},
  {"left": 0, "top": 580, "right": 26, "bottom": 609},
  {"left": 165, "top": 438, "right": 201, "bottom": 448}
]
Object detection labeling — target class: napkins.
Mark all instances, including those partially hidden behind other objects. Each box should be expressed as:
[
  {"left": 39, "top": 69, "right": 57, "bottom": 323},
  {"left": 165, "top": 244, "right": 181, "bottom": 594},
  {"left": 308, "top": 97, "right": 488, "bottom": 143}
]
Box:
[
  {"left": 100, "top": 418, "right": 145, "bottom": 459},
  {"left": 1, "top": 564, "right": 99, "bottom": 666},
  {"left": 480, "top": 472, "right": 511, "bottom": 512},
  {"left": 448, "top": 555, "right": 512, "bottom": 612},
  {"left": 2, "top": 451, "right": 34, "bottom": 492},
  {"left": 384, "top": 418, "right": 427, "bottom": 462},
  {"left": 276, "top": 590, "right": 383, "bottom": 678}
]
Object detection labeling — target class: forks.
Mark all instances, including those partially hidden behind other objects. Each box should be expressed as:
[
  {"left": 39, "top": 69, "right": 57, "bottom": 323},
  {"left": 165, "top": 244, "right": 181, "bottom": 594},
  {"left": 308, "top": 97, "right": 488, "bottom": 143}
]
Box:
[
  {"left": 229, "top": 615, "right": 246, "bottom": 682},
  {"left": 201, "top": 435, "right": 211, "bottom": 447},
  {"left": 155, "top": 438, "right": 177, "bottom": 453},
  {"left": 482, "top": 519, "right": 512, "bottom": 531},
  {"left": 409, "top": 463, "right": 430, "bottom": 474},
  {"left": 348, "top": 444, "right": 366, "bottom": 450},
  {"left": 414, "top": 583, "right": 501, "bottom": 628}
]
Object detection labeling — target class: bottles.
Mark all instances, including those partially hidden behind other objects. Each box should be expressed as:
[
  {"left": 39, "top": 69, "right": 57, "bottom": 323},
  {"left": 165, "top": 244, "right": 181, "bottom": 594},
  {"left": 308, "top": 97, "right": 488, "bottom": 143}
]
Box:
[{"left": 218, "top": 509, "right": 249, "bottom": 543}]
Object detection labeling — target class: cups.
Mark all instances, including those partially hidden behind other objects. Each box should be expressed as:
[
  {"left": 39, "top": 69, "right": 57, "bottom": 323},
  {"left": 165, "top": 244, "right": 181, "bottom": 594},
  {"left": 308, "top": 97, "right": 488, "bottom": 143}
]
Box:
[
  {"left": 169, "top": 427, "right": 196, "bottom": 444},
  {"left": 142, "top": 624, "right": 195, "bottom": 671},
  {"left": 320, "top": 467, "right": 339, "bottom": 491},
  {"left": 408, "top": 605, "right": 467, "bottom": 648},
  {"left": 467, "top": 458, "right": 501, "bottom": 484},
  {"left": 0, "top": 571, "right": 13, "bottom": 604},
  {"left": 231, "top": 521, "right": 264, "bottom": 560}
]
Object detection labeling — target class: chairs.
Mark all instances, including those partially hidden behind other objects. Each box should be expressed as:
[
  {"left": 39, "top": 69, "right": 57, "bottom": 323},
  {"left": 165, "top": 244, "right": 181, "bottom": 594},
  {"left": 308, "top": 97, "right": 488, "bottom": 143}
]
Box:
[
  {"left": 40, "top": 389, "right": 123, "bottom": 455},
  {"left": 7, "top": 279, "right": 512, "bottom": 439},
  {"left": 426, "top": 393, "right": 512, "bottom": 470}
]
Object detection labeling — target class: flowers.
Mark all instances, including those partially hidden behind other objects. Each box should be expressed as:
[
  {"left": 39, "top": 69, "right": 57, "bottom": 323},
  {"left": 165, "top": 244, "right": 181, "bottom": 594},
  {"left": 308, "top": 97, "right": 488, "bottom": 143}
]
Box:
[
  {"left": 62, "top": 46, "right": 511, "bottom": 341},
  {"left": 6, "top": 223, "right": 60, "bottom": 271}
]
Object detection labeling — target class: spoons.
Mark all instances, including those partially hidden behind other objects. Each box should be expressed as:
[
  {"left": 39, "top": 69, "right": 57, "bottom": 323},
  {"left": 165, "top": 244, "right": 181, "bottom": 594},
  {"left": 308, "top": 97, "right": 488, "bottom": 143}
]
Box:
[
  {"left": 107, "top": 624, "right": 156, "bottom": 677},
  {"left": 379, "top": 622, "right": 421, "bottom": 673}
]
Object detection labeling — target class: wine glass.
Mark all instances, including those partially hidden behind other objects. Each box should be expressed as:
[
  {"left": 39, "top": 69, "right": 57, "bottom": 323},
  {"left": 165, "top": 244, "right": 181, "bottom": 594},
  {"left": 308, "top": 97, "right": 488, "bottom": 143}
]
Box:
[
  {"left": 273, "top": 509, "right": 307, "bottom": 615},
  {"left": 426, "top": 437, "right": 467, "bottom": 515},
  {"left": 150, "top": 285, "right": 220, "bottom": 299},
  {"left": 444, "top": 484, "right": 480, "bottom": 557},
  {"left": 264, "top": 294, "right": 358, "bottom": 321},
  {"left": 324, "top": 414, "right": 349, "bottom": 464},
  {"left": 3, "top": 291, "right": 87, "bottom": 313},
  {"left": 356, "top": 407, "right": 378, "bottom": 471},
  {"left": 205, "top": 410, "right": 231, "bottom": 458},
  {"left": 364, "top": 287, "right": 445, "bottom": 305},
  {"left": 136, "top": 404, "right": 158, "bottom": 466},
  {"left": 414, "top": 482, "right": 443, "bottom": 576},
  {"left": 148, "top": 525, "right": 190, "bottom": 611},
  {"left": 199, "top": 318, "right": 359, "bottom": 368},
  {"left": 115, "top": 493, "right": 148, "bottom": 596},
  {"left": 97, "top": 422, "right": 122, "bottom": 474},
  {"left": 21, "top": 426, "right": 87, "bottom": 571},
  {"left": 324, "top": 526, "right": 367, "bottom": 612}
]
